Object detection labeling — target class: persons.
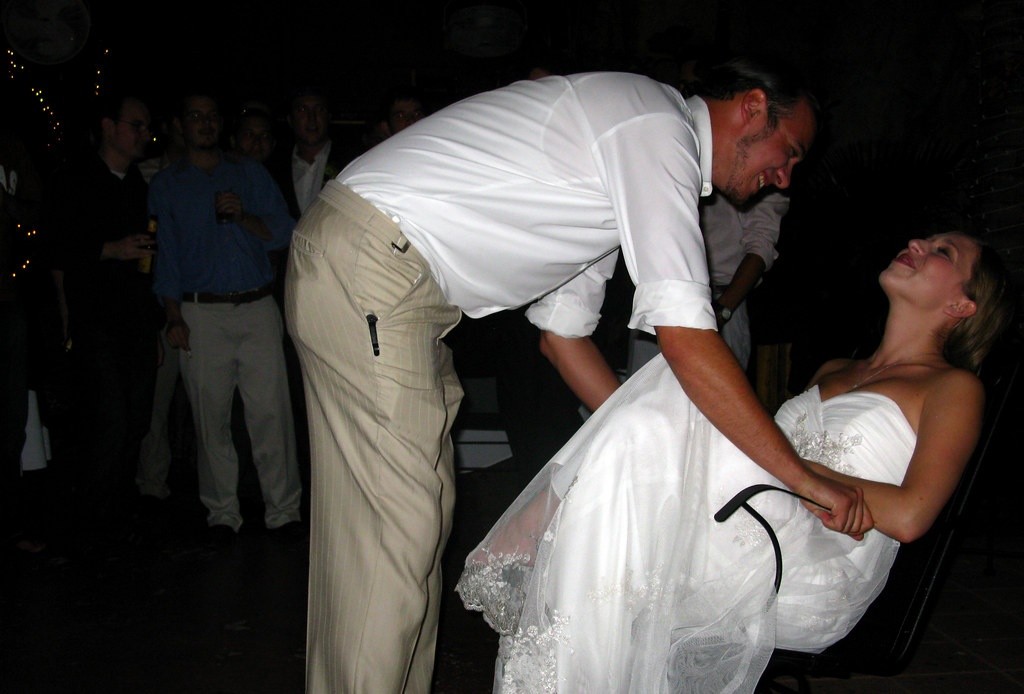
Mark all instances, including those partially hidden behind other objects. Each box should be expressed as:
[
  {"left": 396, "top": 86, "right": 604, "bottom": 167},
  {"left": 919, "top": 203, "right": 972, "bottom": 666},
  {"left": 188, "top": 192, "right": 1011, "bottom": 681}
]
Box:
[
  {"left": 0, "top": 63, "right": 789, "bottom": 547},
  {"left": 278, "top": 54, "right": 874, "bottom": 694},
  {"left": 453, "top": 225, "right": 1023, "bottom": 694}
]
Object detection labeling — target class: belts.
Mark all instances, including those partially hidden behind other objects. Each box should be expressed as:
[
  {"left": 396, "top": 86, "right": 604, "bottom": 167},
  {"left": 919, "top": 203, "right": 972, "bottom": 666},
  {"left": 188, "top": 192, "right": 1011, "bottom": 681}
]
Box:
[{"left": 184, "top": 280, "right": 277, "bottom": 305}]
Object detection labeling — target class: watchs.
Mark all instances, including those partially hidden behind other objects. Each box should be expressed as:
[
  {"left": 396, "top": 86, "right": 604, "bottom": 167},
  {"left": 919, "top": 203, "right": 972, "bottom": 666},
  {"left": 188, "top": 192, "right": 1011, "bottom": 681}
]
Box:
[{"left": 711, "top": 299, "right": 731, "bottom": 322}]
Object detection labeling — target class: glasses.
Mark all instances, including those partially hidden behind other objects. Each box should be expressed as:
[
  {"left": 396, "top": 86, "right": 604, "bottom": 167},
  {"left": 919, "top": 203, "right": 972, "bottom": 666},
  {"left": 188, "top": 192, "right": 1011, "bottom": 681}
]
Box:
[
  {"left": 185, "top": 113, "right": 219, "bottom": 124},
  {"left": 120, "top": 118, "right": 151, "bottom": 132}
]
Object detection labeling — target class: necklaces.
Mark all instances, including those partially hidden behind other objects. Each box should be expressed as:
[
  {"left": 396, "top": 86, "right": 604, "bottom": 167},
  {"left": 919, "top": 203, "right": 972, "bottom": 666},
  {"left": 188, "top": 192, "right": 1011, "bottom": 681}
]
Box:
[{"left": 852, "top": 362, "right": 897, "bottom": 387}]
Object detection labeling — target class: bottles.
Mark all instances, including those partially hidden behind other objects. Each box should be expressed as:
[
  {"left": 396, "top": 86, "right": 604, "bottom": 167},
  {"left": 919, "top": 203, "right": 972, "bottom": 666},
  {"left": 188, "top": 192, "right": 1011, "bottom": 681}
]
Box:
[{"left": 133, "top": 216, "right": 158, "bottom": 281}]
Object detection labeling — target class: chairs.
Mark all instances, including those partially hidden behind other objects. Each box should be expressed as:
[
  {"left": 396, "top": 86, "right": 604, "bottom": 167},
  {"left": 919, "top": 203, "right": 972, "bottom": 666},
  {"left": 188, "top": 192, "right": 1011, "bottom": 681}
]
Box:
[{"left": 714, "top": 336, "right": 1024, "bottom": 694}]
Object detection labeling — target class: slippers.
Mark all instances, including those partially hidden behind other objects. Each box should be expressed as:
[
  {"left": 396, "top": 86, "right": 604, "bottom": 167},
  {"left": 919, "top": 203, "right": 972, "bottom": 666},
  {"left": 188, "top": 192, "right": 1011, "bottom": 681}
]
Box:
[{"left": 0, "top": 530, "right": 50, "bottom": 560}]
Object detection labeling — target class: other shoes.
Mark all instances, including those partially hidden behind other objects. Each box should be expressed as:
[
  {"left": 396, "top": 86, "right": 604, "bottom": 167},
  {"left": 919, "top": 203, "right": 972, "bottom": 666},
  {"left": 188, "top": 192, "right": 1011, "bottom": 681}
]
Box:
[
  {"left": 196, "top": 523, "right": 235, "bottom": 562},
  {"left": 267, "top": 522, "right": 311, "bottom": 555}
]
